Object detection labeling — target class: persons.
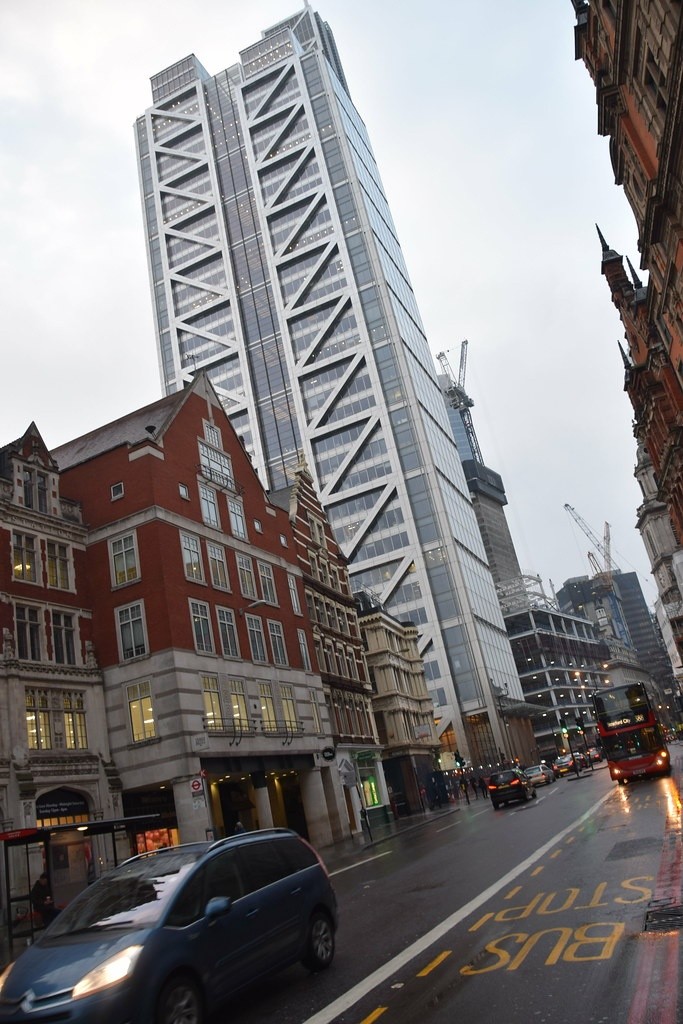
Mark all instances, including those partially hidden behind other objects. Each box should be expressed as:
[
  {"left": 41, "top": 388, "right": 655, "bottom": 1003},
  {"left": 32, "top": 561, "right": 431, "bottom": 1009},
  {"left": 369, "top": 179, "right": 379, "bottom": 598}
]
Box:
[{"left": 460, "top": 774, "right": 489, "bottom": 802}]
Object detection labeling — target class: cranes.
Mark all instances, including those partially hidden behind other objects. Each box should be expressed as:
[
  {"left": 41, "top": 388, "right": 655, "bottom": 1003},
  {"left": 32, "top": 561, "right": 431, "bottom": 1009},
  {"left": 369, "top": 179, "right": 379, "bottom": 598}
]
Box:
[
  {"left": 434, "top": 338, "right": 487, "bottom": 466},
  {"left": 587, "top": 520, "right": 615, "bottom": 596}
]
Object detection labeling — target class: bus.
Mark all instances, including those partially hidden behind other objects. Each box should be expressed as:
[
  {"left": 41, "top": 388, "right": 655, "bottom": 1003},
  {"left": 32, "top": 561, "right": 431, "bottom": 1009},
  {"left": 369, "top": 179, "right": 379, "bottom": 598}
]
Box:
[{"left": 593, "top": 682, "right": 671, "bottom": 784}]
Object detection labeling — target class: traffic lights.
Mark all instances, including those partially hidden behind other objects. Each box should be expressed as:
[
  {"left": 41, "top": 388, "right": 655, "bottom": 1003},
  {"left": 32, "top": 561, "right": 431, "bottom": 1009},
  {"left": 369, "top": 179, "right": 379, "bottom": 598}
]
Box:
[
  {"left": 454, "top": 752, "right": 461, "bottom": 767},
  {"left": 575, "top": 717, "right": 585, "bottom": 732},
  {"left": 560, "top": 719, "right": 567, "bottom": 734}
]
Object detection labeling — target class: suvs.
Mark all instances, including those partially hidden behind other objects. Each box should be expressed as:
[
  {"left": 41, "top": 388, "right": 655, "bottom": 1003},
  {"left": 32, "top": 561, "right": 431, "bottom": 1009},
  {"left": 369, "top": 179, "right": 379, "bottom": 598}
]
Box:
[{"left": 0, "top": 824, "right": 342, "bottom": 1023}]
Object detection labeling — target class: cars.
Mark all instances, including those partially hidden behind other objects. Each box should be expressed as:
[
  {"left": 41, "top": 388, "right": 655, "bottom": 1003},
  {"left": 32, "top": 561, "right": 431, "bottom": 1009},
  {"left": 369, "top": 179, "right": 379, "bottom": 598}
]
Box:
[{"left": 524, "top": 745, "right": 606, "bottom": 786}]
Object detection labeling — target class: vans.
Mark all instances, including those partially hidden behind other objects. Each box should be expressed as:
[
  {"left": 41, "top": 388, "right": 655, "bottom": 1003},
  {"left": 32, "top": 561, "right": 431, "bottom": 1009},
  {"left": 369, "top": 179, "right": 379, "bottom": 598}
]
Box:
[{"left": 488, "top": 769, "right": 537, "bottom": 810}]
